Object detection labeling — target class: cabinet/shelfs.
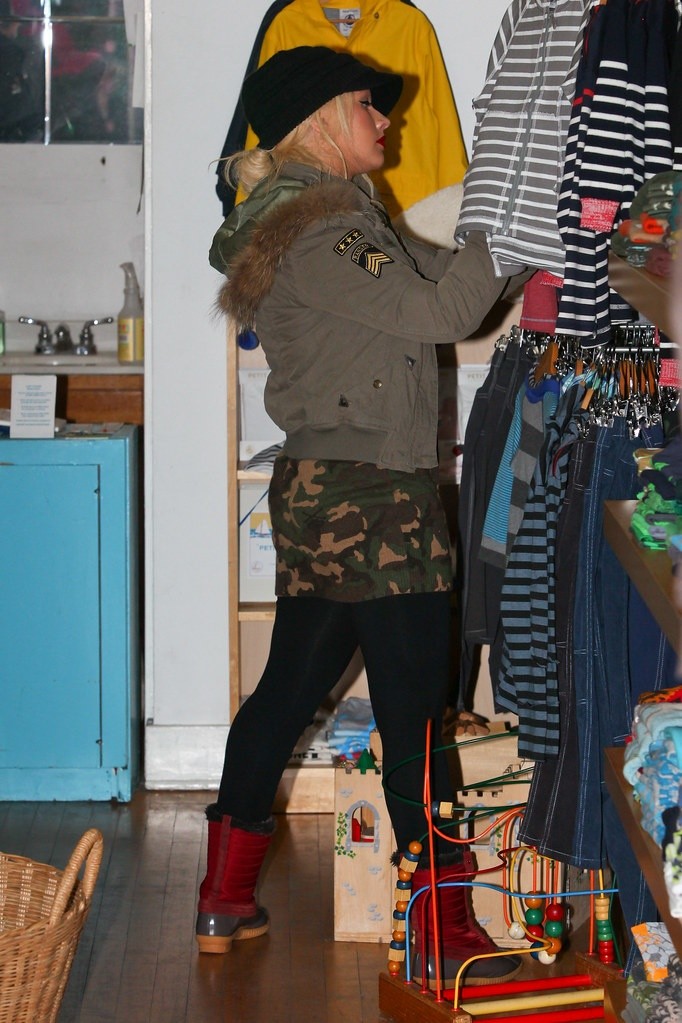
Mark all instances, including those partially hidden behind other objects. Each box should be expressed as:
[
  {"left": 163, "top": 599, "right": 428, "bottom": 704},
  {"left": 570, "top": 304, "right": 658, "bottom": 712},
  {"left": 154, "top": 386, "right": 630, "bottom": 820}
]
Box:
[
  {"left": 226, "top": 298, "right": 527, "bottom": 815},
  {"left": 599, "top": 250, "right": 682, "bottom": 961},
  {"left": 0, "top": 422, "right": 141, "bottom": 804}
]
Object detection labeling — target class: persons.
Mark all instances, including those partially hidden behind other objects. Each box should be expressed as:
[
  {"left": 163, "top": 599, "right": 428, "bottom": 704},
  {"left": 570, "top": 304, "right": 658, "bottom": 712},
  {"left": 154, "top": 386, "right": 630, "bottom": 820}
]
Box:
[{"left": 196, "top": 45, "right": 539, "bottom": 989}]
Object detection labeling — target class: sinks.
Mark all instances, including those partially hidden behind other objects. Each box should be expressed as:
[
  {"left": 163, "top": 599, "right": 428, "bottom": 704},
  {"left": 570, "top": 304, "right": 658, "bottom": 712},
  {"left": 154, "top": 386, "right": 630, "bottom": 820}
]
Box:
[{"left": 0, "top": 357, "right": 99, "bottom": 368}]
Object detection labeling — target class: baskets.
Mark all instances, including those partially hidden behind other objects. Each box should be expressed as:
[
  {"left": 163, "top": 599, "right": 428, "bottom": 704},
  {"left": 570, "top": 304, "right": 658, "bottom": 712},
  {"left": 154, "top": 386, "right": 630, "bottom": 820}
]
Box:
[{"left": 0, "top": 828, "right": 103, "bottom": 1023}]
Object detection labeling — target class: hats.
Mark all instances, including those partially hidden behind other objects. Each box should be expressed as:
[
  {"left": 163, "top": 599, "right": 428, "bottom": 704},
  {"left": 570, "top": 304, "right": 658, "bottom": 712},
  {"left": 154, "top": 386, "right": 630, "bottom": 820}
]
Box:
[{"left": 242, "top": 46, "right": 404, "bottom": 149}]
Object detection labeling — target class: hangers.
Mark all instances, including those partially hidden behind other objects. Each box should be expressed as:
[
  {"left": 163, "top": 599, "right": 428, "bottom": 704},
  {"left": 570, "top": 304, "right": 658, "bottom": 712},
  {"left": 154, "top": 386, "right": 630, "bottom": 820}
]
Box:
[{"left": 493, "top": 322, "right": 681, "bottom": 440}]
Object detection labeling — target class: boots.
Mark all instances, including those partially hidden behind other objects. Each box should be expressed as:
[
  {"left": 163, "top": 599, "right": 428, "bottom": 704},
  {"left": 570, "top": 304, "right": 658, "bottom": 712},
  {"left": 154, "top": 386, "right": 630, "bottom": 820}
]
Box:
[
  {"left": 195, "top": 805, "right": 278, "bottom": 954},
  {"left": 390, "top": 841, "right": 524, "bottom": 990}
]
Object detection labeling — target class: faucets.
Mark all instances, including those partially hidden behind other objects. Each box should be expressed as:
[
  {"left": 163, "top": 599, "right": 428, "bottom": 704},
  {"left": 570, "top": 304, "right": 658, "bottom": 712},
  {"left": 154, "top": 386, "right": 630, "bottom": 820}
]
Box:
[{"left": 55, "top": 324, "right": 73, "bottom": 350}]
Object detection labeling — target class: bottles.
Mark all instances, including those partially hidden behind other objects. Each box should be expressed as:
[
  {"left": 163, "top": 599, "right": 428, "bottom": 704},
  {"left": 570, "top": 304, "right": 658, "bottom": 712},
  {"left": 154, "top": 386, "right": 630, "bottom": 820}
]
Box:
[{"left": 117, "top": 263, "right": 144, "bottom": 366}]
